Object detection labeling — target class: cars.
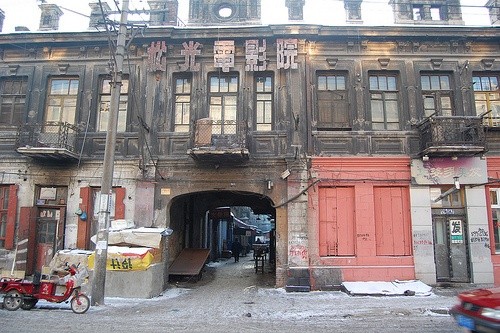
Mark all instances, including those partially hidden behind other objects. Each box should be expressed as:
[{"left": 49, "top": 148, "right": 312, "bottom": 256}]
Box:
[{"left": 451, "top": 287, "right": 500, "bottom": 333}]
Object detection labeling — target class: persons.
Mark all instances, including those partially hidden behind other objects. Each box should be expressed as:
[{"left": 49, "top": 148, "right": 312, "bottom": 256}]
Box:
[{"left": 232, "top": 238, "right": 241, "bottom": 262}]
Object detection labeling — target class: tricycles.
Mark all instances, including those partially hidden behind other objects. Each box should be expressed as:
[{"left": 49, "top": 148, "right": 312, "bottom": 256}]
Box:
[{"left": 0, "top": 262, "right": 90, "bottom": 314}]
